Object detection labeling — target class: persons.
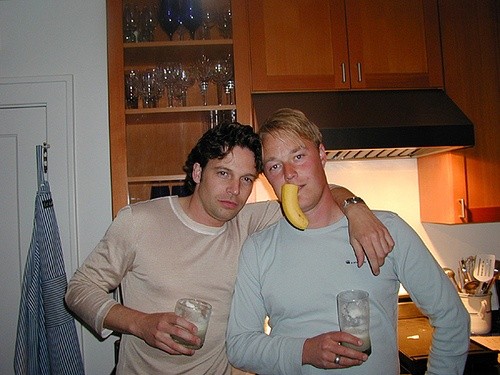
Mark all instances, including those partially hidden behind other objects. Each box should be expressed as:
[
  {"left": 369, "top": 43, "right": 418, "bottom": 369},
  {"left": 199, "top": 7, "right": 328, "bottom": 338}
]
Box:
[
  {"left": 63, "top": 120, "right": 395, "bottom": 375},
  {"left": 225, "top": 108, "right": 471, "bottom": 375}
]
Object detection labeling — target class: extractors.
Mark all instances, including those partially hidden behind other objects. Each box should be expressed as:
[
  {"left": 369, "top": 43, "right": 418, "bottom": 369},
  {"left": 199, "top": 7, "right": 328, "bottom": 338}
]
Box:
[{"left": 250, "top": 88, "right": 474, "bottom": 159}]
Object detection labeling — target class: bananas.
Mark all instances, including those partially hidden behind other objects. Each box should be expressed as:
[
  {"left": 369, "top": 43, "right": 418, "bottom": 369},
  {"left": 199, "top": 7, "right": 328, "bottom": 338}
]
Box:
[{"left": 281, "top": 183, "right": 309, "bottom": 230}]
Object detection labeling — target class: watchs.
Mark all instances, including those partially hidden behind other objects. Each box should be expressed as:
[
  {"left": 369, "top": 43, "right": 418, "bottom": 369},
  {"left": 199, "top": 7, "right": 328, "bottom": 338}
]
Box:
[{"left": 341, "top": 196, "right": 365, "bottom": 211}]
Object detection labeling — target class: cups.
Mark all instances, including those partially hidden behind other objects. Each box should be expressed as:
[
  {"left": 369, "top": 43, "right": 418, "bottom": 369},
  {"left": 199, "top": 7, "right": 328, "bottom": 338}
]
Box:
[
  {"left": 141, "top": 68, "right": 158, "bottom": 109},
  {"left": 125, "top": 69, "right": 142, "bottom": 109},
  {"left": 169, "top": 298, "right": 212, "bottom": 350},
  {"left": 458, "top": 290, "right": 493, "bottom": 335},
  {"left": 337, "top": 289, "right": 372, "bottom": 357}
]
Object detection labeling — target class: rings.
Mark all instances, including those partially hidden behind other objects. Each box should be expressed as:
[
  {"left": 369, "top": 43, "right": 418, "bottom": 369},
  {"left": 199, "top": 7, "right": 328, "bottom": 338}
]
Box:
[{"left": 335, "top": 356, "right": 340, "bottom": 364}]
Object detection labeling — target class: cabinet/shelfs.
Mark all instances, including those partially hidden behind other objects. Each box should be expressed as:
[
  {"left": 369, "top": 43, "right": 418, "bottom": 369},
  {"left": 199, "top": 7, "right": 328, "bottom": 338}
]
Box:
[{"left": 105, "top": 0, "right": 500, "bottom": 228}]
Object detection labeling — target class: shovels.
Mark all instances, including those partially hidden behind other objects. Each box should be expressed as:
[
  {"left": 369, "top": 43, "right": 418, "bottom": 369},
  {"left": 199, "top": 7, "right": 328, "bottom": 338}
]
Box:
[{"left": 472, "top": 254, "right": 495, "bottom": 294}]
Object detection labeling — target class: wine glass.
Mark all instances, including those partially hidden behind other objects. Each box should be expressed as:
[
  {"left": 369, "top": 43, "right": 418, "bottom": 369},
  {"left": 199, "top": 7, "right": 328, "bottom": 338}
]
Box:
[
  {"left": 157, "top": 54, "right": 234, "bottom": 107},
  {"left": 122, "top": 0, "right": 232, "bottom": 43}
]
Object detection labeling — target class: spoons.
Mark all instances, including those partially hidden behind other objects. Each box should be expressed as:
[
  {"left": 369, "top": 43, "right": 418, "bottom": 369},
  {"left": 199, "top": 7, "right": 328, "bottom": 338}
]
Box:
[
  {"left": 443, "top": 268, "right": 462, "bottom": 293},
  {"left": 463, "top": 281, "right": 480, "bottom": 293}
]
